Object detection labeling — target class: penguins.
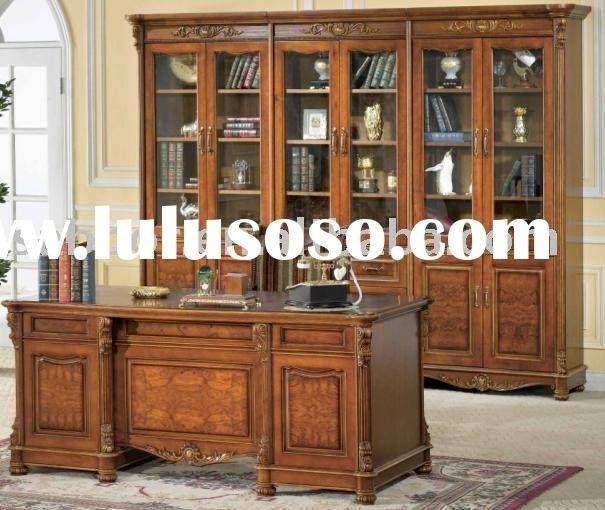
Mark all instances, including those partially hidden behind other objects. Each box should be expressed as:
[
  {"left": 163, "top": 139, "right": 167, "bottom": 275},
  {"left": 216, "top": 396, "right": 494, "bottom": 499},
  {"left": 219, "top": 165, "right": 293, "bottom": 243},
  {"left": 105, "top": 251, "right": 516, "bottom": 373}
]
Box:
[{"left": 425, "top": 147, "right": 457, "bottom": 195}]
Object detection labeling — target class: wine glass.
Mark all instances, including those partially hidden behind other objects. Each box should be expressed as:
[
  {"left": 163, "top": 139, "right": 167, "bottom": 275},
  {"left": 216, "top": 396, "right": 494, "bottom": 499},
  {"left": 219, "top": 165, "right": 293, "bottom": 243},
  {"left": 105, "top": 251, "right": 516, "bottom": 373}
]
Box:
[{"left": 493, "top": 59, "right": 507, "bottom": 89}]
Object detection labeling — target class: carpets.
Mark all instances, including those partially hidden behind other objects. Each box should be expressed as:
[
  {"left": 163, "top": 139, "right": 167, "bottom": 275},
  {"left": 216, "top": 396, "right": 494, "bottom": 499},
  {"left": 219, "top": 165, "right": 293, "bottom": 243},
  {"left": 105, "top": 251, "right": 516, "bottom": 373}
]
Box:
[{"left": 0, "top": 436, "right": 583, "bottom": 510}]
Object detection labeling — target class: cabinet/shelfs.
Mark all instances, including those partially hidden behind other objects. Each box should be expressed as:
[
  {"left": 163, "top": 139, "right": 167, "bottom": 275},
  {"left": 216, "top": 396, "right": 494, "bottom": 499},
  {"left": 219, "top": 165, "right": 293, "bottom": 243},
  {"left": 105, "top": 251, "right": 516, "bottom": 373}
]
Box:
[{"left": 123, "top": 3, "right": 592, "bottom": 400}]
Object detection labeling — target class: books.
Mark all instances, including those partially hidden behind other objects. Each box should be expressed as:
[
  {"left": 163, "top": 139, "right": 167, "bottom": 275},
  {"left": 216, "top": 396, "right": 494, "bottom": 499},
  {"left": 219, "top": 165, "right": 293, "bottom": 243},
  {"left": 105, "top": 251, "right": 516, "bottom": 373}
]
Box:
[
  {"left": 38, "top": 241, "right": 96, "bottom": 305},
  {"left": 156, "top": 50, "right": 537, "bottom": 245}
]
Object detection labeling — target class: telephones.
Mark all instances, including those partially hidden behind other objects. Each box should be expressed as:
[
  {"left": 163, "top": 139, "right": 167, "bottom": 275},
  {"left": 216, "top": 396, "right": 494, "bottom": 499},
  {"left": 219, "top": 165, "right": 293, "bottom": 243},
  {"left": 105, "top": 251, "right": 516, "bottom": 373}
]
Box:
[{"left": 284, "top": 253, "right": 352, "bottom": 312}]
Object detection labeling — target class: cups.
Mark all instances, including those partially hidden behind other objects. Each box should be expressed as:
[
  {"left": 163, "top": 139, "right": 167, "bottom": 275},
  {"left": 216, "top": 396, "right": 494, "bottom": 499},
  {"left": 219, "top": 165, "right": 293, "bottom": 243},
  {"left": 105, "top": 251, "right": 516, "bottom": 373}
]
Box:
[{"left": 197, "top": 265, "right": 213, "bottom": 297}]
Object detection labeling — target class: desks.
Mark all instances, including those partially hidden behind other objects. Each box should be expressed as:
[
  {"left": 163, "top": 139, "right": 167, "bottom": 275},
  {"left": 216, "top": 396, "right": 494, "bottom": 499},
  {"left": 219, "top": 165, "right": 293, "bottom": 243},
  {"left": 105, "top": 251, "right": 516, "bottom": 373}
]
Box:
[{"left": 1, "top": 285, "right": 434, "bottom": 503}]
